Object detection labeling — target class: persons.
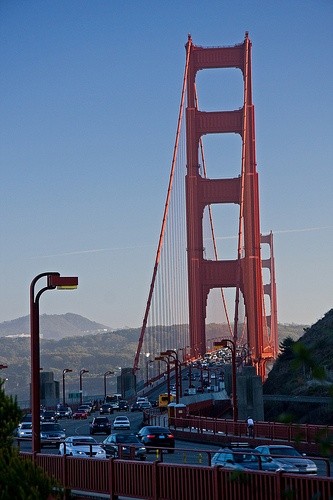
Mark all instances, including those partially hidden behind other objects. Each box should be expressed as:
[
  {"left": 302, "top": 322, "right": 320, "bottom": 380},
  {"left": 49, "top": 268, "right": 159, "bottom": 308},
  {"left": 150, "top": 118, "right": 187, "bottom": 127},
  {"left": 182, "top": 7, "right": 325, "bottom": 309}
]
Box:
[{"left": 247, "top": 416, "right": 255, "bottom": 435}]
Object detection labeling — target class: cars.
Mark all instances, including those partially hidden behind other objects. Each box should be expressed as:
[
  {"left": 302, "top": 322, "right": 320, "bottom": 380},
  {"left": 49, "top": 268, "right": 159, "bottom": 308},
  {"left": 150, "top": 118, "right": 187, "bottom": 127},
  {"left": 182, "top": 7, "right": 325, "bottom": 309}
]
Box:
[
  {"left": 136, "top": 426, "right": 175, "bottom": 454},
  {"left": 89, "top": 417, "right": 111, "bottom": 436},
  {"left": 38, "top": 422, "right": 66, "bottom": 448},
  {"left": 101, "top": 433, "right": 147, "bottom": 461},
  {"left": 40, "top": 401, "right": 97, "bottom": 423},
  {"left": 100, "top": 405, "right": 114, "bottom": 415},
  {"left": 113, "top": 416, "right": 131, "bottom": 430},
  {"left": 18, "top": 421, "right": 33, "bottom": 438},
  {"left": 252, "top": 445, "right": 318, "bottom": 476},
  {"left": 57, "top": 436, "right": 106, "bottom": 460},
  {"left": 118, "top": 345, "right": 243, "bottom": 412},
  {"left": 210, "top": 442, "right": 281, "bottom": 472}
]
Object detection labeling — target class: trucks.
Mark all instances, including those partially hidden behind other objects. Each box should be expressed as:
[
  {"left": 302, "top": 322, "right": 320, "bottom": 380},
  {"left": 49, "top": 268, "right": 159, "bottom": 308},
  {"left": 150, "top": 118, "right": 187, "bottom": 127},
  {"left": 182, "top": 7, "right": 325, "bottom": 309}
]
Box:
[{"left": 159, "top": 393, "right": 176, "bottom": 412}]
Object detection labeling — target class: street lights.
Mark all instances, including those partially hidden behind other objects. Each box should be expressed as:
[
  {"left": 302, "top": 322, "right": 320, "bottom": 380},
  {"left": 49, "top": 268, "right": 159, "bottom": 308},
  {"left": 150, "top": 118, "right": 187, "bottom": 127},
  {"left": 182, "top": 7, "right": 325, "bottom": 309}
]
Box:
[
  {"left": 80, "top": 370, "right": 89, "bottom": 404},
  {"left": 155, "top": 350, "right": 225, "bottom": 404},
  {"left": 104, "top": 371, "right": 114, "bottom": 398},
  {"left": 63, "top": 369, "right": 72, "bottom": 405},
  {"left": 213, "top": 339, "right": 238, "bottom": 435},
  {"left": 30, "top": 272, "right": 78, "bottom": 455}
]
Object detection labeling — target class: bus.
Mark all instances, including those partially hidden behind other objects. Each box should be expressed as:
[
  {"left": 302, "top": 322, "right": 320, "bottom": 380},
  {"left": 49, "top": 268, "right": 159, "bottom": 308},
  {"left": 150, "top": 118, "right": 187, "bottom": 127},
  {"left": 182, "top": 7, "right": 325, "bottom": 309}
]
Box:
[{"left": 104, "top": 394, "right": 121, "bottom": 409}]
